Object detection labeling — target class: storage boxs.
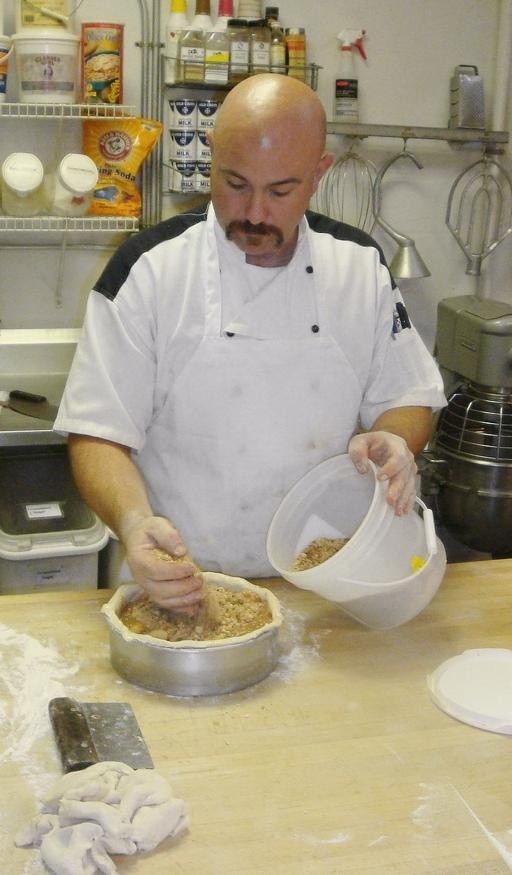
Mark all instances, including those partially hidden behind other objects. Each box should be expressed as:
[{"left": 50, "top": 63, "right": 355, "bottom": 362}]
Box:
[
  {"left": 105, "top": 526, "right": 135, "bottom": 588},
  {"left": 0, "top": 444, "right": 110, "bottom": 595}
]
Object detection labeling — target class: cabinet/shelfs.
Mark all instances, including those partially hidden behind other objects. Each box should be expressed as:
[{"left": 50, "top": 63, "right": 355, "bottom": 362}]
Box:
[
  {"left": 157, "top": 52, "right": 324, "bottom": 197},
  {"left": 0, "top": 101, "right": 143, "bottom": 251}
]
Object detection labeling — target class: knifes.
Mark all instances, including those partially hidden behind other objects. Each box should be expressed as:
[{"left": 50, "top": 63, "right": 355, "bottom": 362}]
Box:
[{"left": 50, "top": 696, "right": 156, "bottom": 771}]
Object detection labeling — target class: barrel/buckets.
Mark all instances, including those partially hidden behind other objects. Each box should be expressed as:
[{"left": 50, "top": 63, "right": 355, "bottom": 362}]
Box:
[
  {"left": 0, "top": 34, "right": 13, "bottom": 102},
  {"left": 265, "top": 452, "right": 446, "bottom": 631},
  {"left": 11, "top": 29, "right": 80, "bottom": 104}
]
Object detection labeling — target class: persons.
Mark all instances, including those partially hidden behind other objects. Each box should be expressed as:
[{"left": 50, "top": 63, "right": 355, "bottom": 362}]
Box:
[{"left": 50, "top": 71, "right": 450, "bottom": 616}]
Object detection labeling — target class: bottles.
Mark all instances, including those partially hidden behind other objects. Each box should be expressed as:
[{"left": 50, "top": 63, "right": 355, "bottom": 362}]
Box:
[
  {"left": 331, "top": 46, "right": 360, "bottom": 123},
  {"left": 43, "top": 155, "right": 98, "bottom": 215},
  {"left": 1, "top": 152, "right": 55, "bottom": 218},
  {"left": 166, "top": 96, "right": 221, "bottom": 194},
  {"left": 165, "top": 0, "right": 309, "bottom": 88}
]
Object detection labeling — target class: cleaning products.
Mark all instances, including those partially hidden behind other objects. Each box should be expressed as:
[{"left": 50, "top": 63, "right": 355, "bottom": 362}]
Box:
[{"left": 331, "top": 28, "right": 368, "bottom": 124}]
